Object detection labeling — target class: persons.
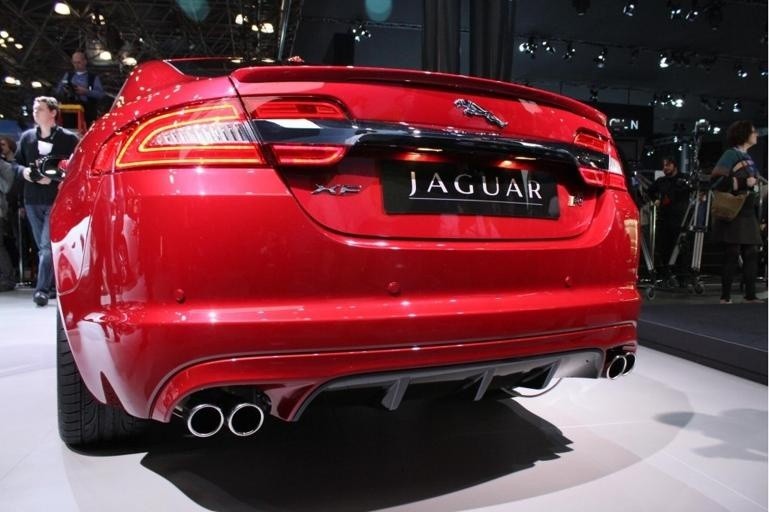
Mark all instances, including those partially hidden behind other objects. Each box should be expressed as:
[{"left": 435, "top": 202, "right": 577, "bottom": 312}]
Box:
[
  {"left": 645, "top": 152, "right": 709, "bottom": 289},
  {"left": 1, "top": 51, "right": 107, "bottom": 307},
  {"left": 705, "top": 117, "right": 767, "bottom": 306}
]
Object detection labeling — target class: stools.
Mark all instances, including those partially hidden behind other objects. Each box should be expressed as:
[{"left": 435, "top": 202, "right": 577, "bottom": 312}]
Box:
[{"left": 57, "top": 104, "right": 87, "bottom": 134}]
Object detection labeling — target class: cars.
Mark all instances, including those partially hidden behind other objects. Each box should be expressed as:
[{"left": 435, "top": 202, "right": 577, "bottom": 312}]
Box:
[{"left": 48, "top": 55, "right": 642, "bottom": 448}]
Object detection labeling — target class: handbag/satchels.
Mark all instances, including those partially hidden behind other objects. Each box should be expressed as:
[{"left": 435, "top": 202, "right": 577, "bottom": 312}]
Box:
[{"left": 709, "top": 187, "right": 748, "bottom": 221}]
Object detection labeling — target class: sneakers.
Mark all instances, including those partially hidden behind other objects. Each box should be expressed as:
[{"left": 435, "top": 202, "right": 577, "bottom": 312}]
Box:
[{"left": 32, "top": 291, "right": 47, "bottom": 306}]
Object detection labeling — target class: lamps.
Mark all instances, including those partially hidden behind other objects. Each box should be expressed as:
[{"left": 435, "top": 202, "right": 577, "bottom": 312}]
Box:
[
  {"left": 352, "top": 26, "right": 371, "bottom": 41},
  {"left": 519, "top": 0, "right": 768, "bottom": 113}
]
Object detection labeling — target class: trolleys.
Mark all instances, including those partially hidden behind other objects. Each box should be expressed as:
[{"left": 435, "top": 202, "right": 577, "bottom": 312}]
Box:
[{"left": 635, "top": 189, "right": 711, "bottom": 302}]
